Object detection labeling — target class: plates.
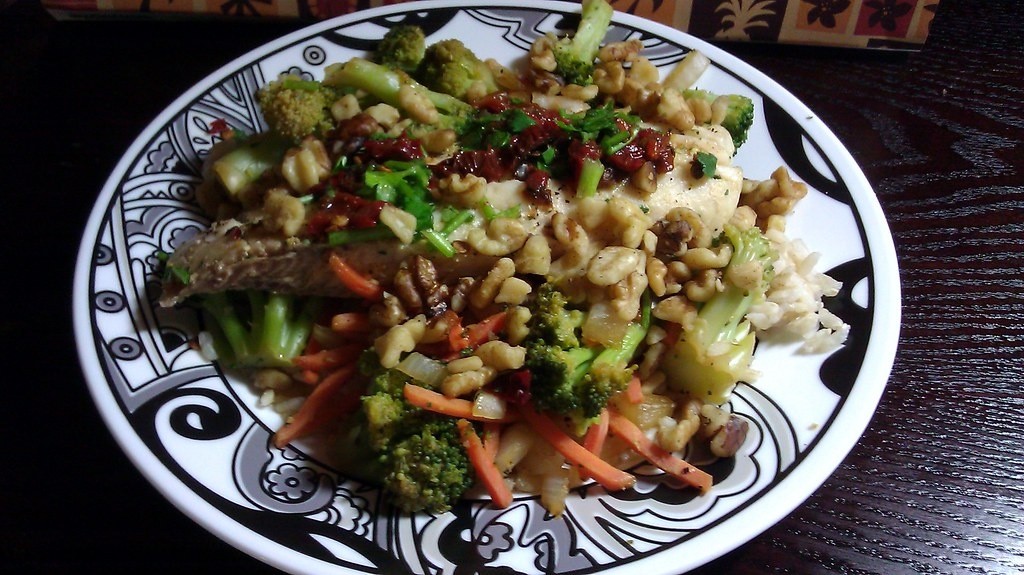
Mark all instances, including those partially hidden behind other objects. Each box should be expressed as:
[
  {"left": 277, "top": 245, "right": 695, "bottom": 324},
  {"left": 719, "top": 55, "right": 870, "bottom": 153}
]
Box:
[{"left": 73, "top": 0, "right": 900, "bottom": 575}]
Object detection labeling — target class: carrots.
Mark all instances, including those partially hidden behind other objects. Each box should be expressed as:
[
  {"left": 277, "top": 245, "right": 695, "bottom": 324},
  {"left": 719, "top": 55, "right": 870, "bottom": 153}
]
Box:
[{"left": 270, "top": 254, "right": 714, "bottom": 509}]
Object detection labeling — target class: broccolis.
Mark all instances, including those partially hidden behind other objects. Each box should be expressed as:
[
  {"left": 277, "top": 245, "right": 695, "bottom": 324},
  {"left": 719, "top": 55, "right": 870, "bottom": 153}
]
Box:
[
  {"left": 208, "top": 0, "right": 778, "bottom": 438},
  {"left": 345, "top": 346, "right": 480, "bottom": 513}
]
